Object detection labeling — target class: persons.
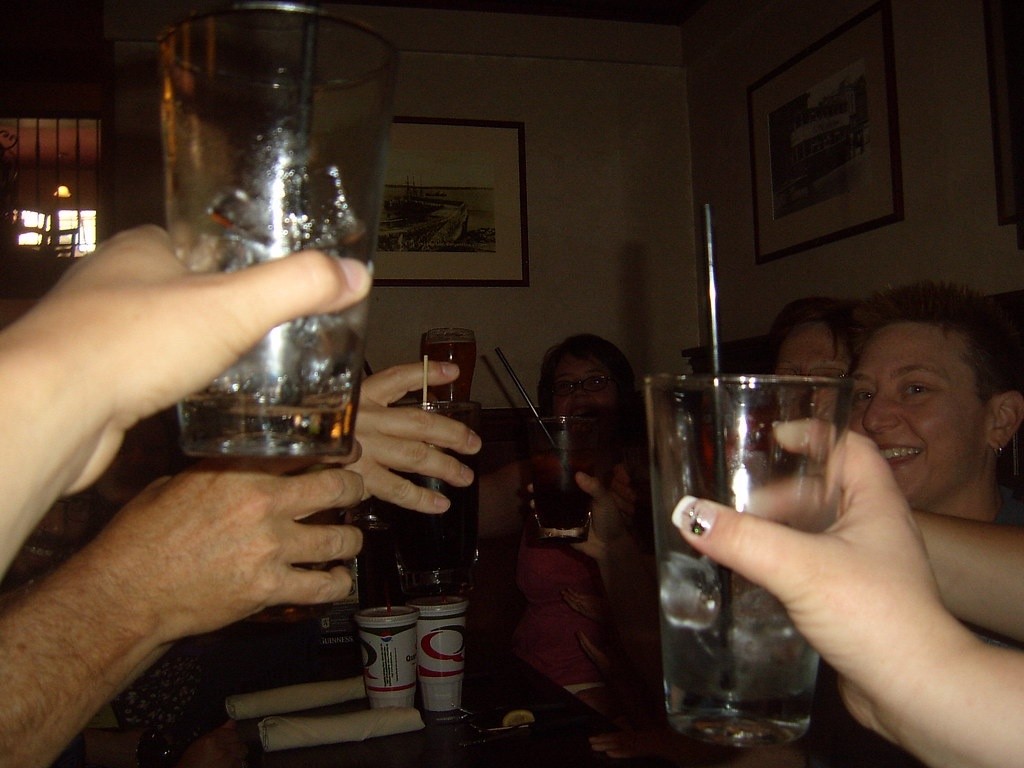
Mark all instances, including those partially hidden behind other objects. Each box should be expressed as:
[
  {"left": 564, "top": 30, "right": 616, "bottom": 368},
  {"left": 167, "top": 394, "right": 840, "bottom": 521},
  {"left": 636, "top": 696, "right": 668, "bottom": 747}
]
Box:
[{"left": 0, "top": 224, "right": 1024, "bottom": 766}]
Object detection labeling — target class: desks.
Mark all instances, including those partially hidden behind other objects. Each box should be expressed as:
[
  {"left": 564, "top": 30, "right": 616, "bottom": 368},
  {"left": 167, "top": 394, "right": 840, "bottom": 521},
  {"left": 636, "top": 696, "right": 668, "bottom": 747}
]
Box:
[{"left": 238, "top": 625, "right": 678, "bottom": 768}]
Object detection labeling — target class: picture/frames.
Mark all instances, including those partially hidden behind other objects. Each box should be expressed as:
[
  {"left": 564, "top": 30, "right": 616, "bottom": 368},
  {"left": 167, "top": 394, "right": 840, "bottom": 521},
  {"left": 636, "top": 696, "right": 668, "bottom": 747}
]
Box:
[
  {"left": 744, "top": 1, "right": 906, "bottom": 266},
  {"left": 371, "top": 115, "right": 530, "bottom": 287}
]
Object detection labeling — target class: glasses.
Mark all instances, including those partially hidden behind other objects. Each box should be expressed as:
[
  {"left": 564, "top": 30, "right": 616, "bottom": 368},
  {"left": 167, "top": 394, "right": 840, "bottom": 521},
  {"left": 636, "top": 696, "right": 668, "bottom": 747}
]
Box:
[
  {"left": 774, "top": 367, "right": 849, "bottom": 379},
  {"left": 549, "top": 375, "right": 619, "bottom": 397}
]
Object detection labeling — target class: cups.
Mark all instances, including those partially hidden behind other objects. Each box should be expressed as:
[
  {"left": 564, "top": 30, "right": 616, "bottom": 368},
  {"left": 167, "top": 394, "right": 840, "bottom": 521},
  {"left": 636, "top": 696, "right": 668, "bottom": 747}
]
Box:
[
  {"left": 155, "top": 3, "right": 398, "bottom": 474},
  {"left": 644, "top": 374, "right": 853, "bottom": 747},
  {"left": 353, "top": 607, "right": 420, "bottom": 709},
  {"left": 421, "top": 327, "right": 476, "bottom": 403},
  {"left": 528, "top": 415, "right": 601, "bottom": 541},
  {"left": 416, "top": 599, "right": 468, "bottom": 712},
  {"left": 389, "top": 402, "right": 482, "bottom": 597}
]
